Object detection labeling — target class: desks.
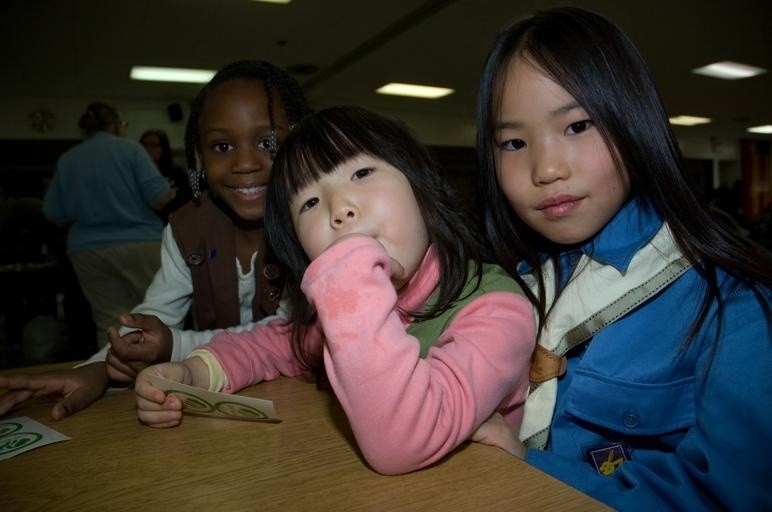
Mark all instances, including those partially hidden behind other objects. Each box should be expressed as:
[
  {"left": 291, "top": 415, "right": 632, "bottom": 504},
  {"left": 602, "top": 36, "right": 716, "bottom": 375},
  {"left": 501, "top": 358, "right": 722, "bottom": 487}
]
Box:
[{"left": 0, "top": 357, "right": 619, "bottom": 511}]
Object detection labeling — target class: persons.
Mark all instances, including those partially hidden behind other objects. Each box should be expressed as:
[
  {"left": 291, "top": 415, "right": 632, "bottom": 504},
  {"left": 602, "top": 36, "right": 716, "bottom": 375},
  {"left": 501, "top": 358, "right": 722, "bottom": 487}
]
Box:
[
  {"left": 0, "top": 60, "right": 302, "bottom": 420},
  {"left": 471, "top": 2, "right": 772, "bottom": 511},
  {"left": 135, "top": 104, "right": 536, "bottom": 476},
  {"left": 1, "top": 102, "right": 189, "bottom": 350}
]
